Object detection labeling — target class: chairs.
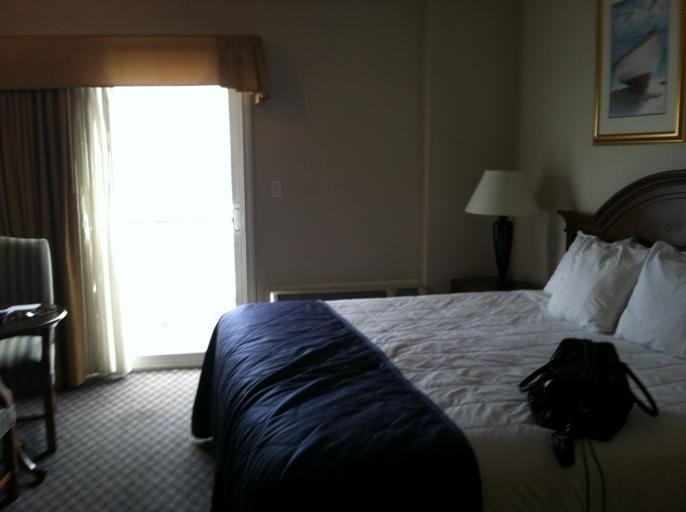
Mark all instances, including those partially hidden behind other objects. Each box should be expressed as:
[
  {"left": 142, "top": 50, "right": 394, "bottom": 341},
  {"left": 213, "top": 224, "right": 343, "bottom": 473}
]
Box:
[{"left": 0, "top": 235, "right": 59, "bottom": 424}]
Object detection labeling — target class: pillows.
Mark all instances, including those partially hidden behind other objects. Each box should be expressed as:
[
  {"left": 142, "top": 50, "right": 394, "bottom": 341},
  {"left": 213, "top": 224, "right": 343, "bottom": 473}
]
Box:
[{"left": 543, "top": 229, "right": 684, "bottom": 359}]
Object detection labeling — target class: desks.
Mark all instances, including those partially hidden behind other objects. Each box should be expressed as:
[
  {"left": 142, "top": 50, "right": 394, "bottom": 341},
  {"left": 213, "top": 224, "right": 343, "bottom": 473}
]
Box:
[{"left": 0, "top": 301, "right": 68, "bottom": 454}]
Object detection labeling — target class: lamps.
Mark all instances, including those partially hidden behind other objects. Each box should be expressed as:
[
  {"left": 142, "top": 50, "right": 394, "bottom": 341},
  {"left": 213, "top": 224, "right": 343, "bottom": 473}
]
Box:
[{"left": 463, "top": 168, "right": 542, "bottom": 291}]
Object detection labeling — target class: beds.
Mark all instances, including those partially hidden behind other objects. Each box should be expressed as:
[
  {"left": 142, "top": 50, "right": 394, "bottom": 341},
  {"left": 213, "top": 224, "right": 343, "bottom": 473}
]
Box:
[{"left": 207, "top": 167, "right": 685, "bottom": 511}]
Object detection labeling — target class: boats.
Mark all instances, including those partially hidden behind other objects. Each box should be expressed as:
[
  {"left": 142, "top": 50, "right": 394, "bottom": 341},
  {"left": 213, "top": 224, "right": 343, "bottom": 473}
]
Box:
[{"left": 615, "top": 26, "right": 660, "bottom": 92}]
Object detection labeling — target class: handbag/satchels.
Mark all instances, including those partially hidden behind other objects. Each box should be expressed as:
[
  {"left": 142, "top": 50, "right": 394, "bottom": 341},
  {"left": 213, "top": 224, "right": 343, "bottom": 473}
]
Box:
[{"left": 519, "top": 337, "right": 659, "bottom": 443}]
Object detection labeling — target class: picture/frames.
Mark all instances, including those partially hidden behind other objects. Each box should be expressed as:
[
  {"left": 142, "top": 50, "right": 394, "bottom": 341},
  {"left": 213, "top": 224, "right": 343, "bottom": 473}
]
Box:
[{"left": 592, "top": 1, "right": 686, "bottom": 148}]
